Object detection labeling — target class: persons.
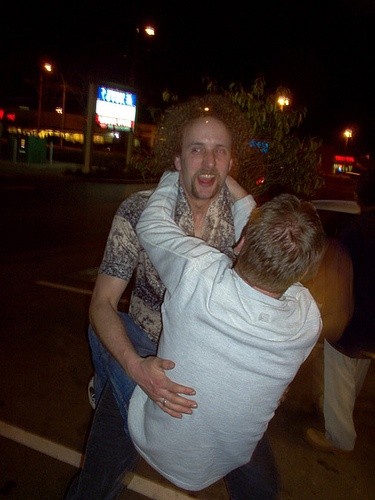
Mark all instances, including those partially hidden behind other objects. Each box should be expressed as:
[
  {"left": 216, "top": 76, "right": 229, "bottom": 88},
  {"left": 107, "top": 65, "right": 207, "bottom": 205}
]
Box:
[
  {"left": 303, "top": 170, "right": 375, "bottom": 457},
  {"left": 75, "top": 92, "right": 288, "bottom": 500},
  {"left": 86, "top": 193, "right": 329, "bottom": 493}
]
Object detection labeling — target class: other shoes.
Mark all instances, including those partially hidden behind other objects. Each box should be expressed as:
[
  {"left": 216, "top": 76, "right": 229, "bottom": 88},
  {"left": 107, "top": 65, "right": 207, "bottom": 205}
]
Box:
[
  {"left": 305, "top": 426, "right": 355, "bottom": 459},
  {"left": 88, "top": 377, "right": 97, "bottom": 410}
]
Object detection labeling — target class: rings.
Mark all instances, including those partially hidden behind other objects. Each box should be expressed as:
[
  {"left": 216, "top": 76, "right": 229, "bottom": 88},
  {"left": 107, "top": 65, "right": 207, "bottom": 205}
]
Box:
[{"left": 162, "top": 398, "right": 168, "bottom": 408}]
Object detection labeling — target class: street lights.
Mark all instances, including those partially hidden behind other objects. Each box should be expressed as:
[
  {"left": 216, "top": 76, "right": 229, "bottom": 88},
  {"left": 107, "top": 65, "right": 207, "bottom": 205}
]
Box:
[{"left": 43, "top": 62, "right": 65, "bottom": 147}]
